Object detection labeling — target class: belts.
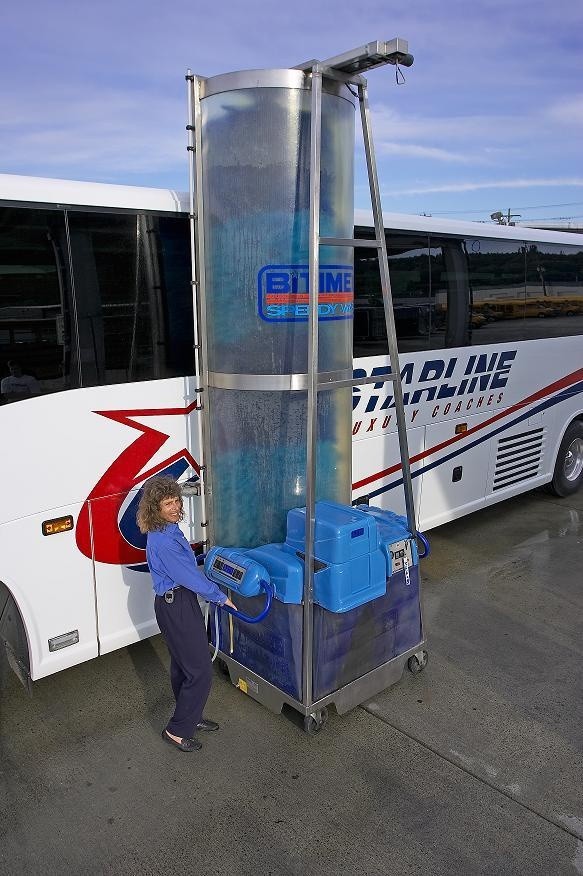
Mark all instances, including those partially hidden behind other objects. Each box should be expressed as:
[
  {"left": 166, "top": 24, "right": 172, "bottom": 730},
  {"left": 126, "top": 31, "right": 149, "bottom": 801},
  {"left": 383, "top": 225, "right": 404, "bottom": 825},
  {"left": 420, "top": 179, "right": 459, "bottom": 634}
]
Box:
[{"left": 168, "top": 585, "right": 181, "bottom": 593}]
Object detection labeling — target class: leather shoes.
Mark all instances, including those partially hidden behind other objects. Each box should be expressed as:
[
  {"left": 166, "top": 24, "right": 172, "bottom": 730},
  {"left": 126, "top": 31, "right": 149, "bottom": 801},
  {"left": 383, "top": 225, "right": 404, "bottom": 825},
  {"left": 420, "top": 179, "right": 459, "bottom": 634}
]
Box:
[
  {"left": 194, "top": 718, "right": 219, "bottom": 731},
  {"left": 161, "top": 729, "right": 202, "bottom": 752}
]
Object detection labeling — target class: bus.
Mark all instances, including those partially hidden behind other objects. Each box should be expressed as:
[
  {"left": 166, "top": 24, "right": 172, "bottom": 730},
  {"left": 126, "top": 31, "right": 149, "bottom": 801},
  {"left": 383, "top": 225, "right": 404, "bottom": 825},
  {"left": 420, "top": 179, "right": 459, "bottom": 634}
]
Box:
[
  {"left": 1, "top": 175, "right": 583, "bottom": 695},
  {"left": 439, "top": 296, "right": 583, "bottom": 329}
]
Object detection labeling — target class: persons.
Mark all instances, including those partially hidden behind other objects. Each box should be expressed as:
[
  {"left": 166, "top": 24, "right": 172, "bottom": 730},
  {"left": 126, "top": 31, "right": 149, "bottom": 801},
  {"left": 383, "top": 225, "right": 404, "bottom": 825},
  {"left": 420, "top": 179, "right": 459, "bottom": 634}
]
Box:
[{"left": 136, "top": 477, "right": 238, "bottom": 754}]
